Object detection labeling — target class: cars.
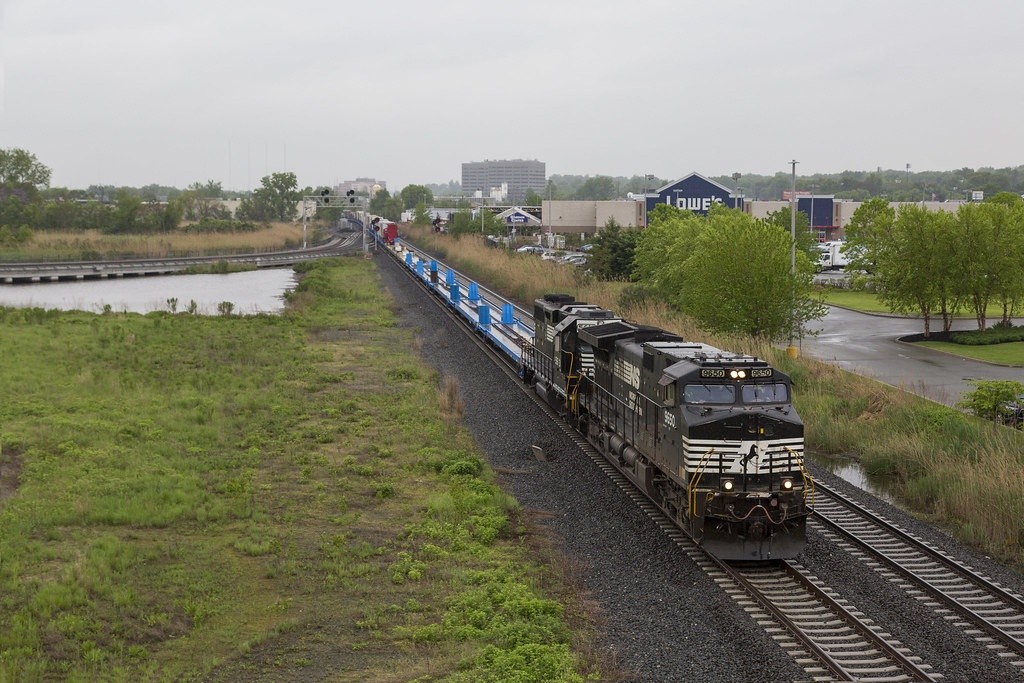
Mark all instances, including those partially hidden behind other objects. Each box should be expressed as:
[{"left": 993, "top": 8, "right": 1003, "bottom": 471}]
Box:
[{"left": 515, "top": 242, "right": 601, "bottom": 267}]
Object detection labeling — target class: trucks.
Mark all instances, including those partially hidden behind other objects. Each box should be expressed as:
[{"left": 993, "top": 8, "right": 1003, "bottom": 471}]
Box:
[{"left": 812, "top": 240, "right": 870, "bottom": 274}]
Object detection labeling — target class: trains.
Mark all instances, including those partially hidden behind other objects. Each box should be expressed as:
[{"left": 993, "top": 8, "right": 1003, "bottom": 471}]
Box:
[
  {"left": 515, "top": 292, "right": 816, "bottom": 567},
  {"left": 340, "top": 209, "right": 399, "bottom": 246}
]
